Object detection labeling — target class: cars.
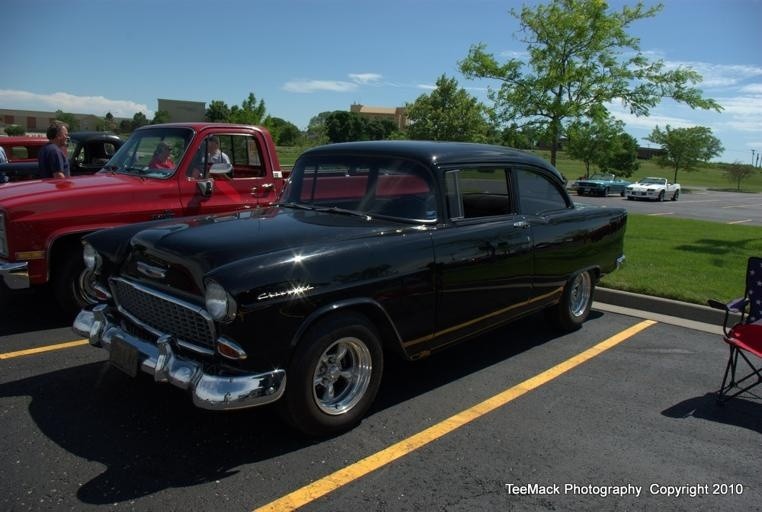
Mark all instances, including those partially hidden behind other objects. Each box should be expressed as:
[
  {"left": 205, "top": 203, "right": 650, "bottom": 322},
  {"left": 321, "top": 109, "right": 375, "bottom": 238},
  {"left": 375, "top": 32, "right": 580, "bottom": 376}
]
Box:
[{"left": 67, "top": 134, "right": 631, "bottom": 445}]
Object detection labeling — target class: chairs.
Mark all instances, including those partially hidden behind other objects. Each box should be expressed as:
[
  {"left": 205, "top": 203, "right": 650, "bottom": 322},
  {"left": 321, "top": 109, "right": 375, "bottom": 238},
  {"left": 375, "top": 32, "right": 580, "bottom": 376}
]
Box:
[{"left": 708, "top": 256, "right": 762, "bottom": 407}]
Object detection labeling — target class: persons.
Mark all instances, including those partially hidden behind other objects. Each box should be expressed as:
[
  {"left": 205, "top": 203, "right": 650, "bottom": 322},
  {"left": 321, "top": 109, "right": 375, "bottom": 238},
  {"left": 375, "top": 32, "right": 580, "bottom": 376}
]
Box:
[
  {"left": 38, "top": 120, "right": 72, "bottom": 179},
  {"left": 149, "top": 141, "right": 176, "bottom": 171},
  {"left": 200, "top": 134, "right": 232, "bottom": 166}
]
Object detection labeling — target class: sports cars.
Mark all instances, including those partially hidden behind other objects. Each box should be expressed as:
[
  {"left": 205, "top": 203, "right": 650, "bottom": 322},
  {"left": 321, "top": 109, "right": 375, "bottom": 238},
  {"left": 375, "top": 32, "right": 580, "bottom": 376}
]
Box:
[
  {"left": 623, "top": 173, "right": 683, "bottom": 203},
  {"left": 569, "top": 168, "right": 629, "bottom": 198}
]
